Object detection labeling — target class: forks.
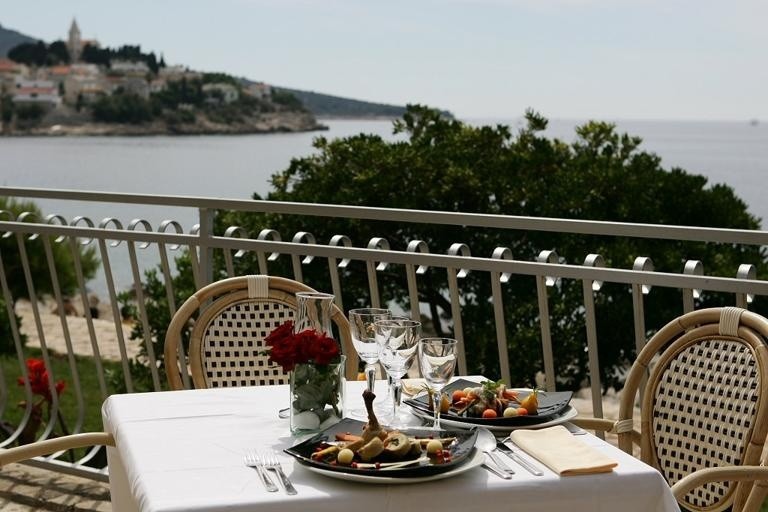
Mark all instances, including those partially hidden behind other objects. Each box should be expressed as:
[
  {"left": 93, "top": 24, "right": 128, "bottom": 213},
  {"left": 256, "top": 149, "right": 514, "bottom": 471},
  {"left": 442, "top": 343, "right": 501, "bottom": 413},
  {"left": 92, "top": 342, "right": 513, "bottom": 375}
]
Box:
[
  {"left": 243, "top": 447, "right": 279, "bottom": 492},
  {"left": 261, "top": 449, "right": 298, "bottom": 496}
]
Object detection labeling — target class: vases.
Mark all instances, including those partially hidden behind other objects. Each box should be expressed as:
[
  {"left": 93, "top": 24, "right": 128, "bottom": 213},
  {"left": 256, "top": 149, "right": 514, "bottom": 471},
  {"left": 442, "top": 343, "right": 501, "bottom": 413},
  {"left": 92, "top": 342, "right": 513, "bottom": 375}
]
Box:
[{"left": 285, "top": 358, "right": 348, "bottom": 433}]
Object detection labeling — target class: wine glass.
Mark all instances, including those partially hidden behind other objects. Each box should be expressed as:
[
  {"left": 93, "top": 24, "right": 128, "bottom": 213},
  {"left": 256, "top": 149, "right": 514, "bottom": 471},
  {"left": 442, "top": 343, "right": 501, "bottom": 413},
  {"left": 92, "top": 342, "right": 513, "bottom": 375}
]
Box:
[
  {"left": 348, "top": 308, "right": 392, "bottom": 418},
  {"left": 373, "top": 319, "right": 423, "bottom": 431},
  {"left": 417, "top": 337, "right": 459, "bottom": 430}
]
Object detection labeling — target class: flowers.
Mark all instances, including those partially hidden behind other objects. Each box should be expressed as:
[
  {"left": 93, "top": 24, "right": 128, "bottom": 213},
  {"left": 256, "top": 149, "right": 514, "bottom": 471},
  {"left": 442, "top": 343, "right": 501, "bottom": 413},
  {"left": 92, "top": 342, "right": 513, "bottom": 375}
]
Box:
[
  {"left": 18, "top": 358, "right": 78, "bottom": 463},
  {"left": 260, "top": 323, "right": 346, "bottom": 421}
]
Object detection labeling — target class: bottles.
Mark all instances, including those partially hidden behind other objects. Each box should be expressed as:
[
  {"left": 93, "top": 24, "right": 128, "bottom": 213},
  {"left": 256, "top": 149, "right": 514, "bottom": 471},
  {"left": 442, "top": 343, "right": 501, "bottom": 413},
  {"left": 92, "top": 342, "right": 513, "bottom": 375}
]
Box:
[{"left": 294, "top": 290, "right": 336, "bottom": 362}]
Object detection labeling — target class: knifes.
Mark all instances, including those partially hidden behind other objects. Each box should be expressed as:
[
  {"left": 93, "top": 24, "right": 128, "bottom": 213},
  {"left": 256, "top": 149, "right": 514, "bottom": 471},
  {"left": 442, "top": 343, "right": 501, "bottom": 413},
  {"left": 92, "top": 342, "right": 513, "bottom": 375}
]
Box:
[{"left": 494, "top": 437, "right": 545, "bottom": 477}]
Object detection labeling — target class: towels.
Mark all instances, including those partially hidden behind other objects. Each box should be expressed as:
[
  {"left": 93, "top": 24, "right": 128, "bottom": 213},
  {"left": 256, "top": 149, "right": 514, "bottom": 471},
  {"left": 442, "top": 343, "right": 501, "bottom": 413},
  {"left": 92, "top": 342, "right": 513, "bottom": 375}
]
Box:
[{"left": 505, "top": 421, "right": 618, "bottom": 475}]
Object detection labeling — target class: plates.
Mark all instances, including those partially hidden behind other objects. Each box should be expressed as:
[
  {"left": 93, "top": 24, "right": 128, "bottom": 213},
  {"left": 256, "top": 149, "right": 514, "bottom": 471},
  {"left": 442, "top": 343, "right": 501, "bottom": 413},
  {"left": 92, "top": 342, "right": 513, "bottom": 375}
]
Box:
[
  {"left": 408, "top": 403, "right": 577, "bottom": 431},
  {"left": 401, "top": 377, "right": 574, "bottom": 424},
  {"left": 295, "top": 446, "right": 485, "bottom": 485},
  {"left": 281, "top": 417, "right": 480, "bottom": 476}
]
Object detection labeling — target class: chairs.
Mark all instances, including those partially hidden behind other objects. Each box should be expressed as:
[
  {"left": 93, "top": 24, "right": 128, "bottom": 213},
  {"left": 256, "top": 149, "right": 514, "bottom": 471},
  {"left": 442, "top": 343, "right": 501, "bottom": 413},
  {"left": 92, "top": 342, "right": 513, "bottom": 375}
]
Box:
[
  {"left": 1, "top": 431, "right": 117, "bottom": 512},
  {"left": 161, "top": 274, "right": 357, "bottom": 390},
  {"left": 566, "top": 307, "right": 767, "bottom": 511}
]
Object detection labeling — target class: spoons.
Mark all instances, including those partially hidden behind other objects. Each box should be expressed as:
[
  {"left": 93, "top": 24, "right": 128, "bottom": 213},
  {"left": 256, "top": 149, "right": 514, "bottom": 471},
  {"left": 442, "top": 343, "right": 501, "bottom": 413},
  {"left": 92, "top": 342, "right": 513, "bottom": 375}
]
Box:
[{"left": 469, "top": 425, "right": 514, "bottom": 475}]
